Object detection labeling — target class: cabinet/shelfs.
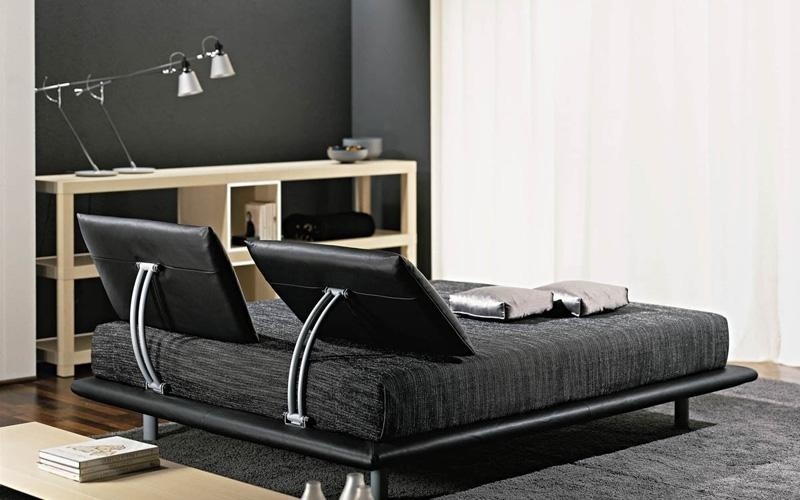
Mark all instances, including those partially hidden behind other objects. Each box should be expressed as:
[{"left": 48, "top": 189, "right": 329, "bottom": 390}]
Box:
[{"left": 33, "top": 154, "right": 419, "bottom": 377}]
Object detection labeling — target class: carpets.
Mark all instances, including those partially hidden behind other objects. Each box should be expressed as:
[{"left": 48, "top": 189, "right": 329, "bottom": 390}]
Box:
[{"left": 102, "top": 377, "right": 798, "bottom": 498}]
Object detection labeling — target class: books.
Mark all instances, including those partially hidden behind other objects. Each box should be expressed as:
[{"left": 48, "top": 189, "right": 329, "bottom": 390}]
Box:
[
  {"left": 244, "top": 201, "right": 278, "bottom": 240},
  {"left": 39, "top": 435, "right": 160, "bottom": 470},
  {"left": 39, "top": 454, "right": 160, "bottom": 475},
  {"left": 37, "top": 459, "right": 161, "bottom": 482}
]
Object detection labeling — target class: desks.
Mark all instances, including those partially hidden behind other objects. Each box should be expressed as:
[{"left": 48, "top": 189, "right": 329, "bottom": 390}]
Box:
[{"left": 1, "top": 419, "right": 293, "bottom": 499}]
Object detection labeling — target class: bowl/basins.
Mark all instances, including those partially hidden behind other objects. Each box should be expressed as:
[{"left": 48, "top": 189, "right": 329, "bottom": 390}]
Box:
[
  {"left": 326, "top": 145, "right": 370, "bottom": 164},
  {"left": 342, "top": 136, "right": 384, "bottom": 161}
]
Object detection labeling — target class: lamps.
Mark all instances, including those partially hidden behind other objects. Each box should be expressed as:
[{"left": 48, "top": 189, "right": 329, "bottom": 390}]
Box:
[{"left": 33, "top": 33, "right": 237, "bottom": 176}]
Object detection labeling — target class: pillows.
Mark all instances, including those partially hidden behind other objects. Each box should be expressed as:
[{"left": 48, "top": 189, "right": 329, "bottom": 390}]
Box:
[
  {"left": 447, "top": 279, "right": 633, "bottom": 323},
  {"left": 76, "top": 213, "right": 255, "bottom": 341},
  {"left": 244, "top": 236, "right": 474, "bottom": 356}
]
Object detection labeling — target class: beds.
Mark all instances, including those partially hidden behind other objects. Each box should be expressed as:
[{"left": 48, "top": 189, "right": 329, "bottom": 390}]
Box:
[{"left": 68, "top": 278, "right": 759, "bottom": 498}]
